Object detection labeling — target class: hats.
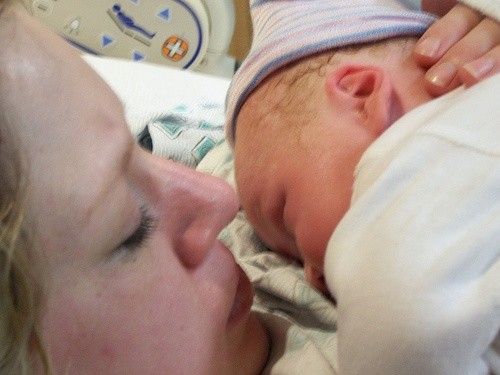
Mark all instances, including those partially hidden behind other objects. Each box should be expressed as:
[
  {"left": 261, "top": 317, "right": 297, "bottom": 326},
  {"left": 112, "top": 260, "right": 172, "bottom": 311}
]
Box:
[{"left": 225, "top": 0, "right": 440, "bottom": 153}]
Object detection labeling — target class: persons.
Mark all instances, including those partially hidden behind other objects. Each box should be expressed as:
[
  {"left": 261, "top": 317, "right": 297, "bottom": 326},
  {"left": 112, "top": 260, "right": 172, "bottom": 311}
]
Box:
[
  {"left": 225, "top": 0, "right": 500, "bottom": 375},
  {"left": 0, "top": 0, "right": 500, "bottom": 375}
]
